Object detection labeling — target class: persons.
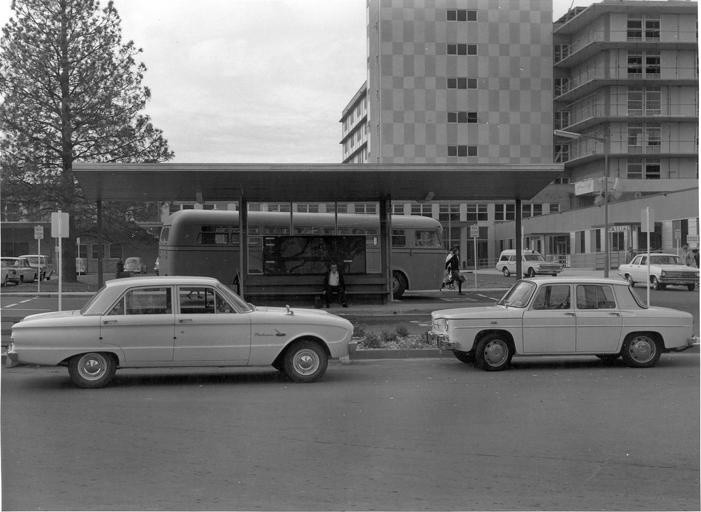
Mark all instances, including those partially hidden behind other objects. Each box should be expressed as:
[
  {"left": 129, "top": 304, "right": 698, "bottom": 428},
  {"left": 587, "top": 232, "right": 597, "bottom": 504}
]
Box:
[
  {"left": 441, "top": 246, "right": 466, "bottom": 296},
  {"left": 154, "top": 255, "right": 159, "bottom": 275},
  {"left": 115, "top": 256, "right": 124, "bottom": 274},
  {"left": 321, "top": 262, "right": 348, "bottom": 308}
]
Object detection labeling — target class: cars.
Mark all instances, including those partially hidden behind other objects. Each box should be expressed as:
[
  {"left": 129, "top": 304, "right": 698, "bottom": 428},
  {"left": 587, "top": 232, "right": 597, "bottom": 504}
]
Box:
[
  {"left": 1, "top": 254, "right": 54, "bottom": 287},
  {"left": 617, "top": 253, "right": 699, "bottom": 292},
  {"left": 4, "top": 274, "right": 356, "bottom": 392},
  {"left": 494, "top": 248, "right": 560, "bottom": 278},
  {"left": 425, "top": 273, "right": 695, "bottom": 369},
  {"left": 123, "top": 256, "right": 148, "bottom": 274},
  {"left": 153, "top": 254, "right": 159, "bottom": 275}
]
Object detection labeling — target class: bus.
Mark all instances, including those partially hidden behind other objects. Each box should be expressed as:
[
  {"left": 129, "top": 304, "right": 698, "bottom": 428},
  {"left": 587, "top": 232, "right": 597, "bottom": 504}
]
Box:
[{"left": 156, "top": 208, "right": 448, "bottom": 301}]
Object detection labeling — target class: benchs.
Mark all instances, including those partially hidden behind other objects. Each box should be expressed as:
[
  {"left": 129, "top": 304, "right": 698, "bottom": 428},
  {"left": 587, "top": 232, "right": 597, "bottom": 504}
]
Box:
[{"left": 242, "top": 274, "right": 390, "bottom": 307}]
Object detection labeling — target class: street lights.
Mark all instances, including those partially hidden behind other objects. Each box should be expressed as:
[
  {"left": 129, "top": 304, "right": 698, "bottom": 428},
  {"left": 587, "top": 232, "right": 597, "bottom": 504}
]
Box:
[{"left": 552, "top": 126, "right": 610, "bottom": 278}]
